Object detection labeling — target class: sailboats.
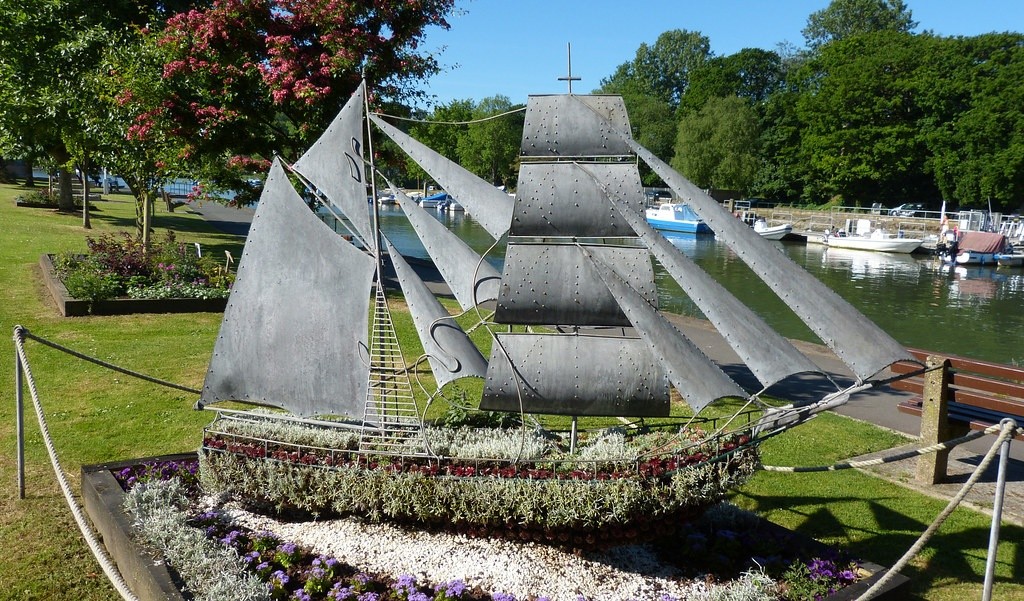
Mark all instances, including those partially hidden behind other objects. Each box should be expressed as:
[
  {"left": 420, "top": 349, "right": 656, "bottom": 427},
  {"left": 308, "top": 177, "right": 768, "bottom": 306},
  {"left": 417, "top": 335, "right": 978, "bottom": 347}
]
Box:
[{"left": 194, "top": 38, "right": 955, "bottom": 538}]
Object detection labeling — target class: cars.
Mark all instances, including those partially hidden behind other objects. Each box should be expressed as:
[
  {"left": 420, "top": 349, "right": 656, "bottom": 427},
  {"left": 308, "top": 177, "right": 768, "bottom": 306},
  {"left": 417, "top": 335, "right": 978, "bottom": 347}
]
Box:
[
  {"left": 740, "top": 197, "right": 776, "bottom": 208},
  {"left": 645, "top": 190, "right": 672, "bottom": 202}
]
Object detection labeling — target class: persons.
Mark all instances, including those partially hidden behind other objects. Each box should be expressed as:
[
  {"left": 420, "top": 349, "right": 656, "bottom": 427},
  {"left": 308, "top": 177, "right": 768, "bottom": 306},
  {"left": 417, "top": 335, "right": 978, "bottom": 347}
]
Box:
[{"left": 939, "top": 215, "right": 950, "bottom": 243}]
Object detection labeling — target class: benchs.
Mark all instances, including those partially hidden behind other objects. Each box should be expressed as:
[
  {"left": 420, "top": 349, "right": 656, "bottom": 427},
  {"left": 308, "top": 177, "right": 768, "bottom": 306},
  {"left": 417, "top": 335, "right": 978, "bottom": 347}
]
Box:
[{"left": 891, "top": 347, "right": 1024, "bottom": 445}]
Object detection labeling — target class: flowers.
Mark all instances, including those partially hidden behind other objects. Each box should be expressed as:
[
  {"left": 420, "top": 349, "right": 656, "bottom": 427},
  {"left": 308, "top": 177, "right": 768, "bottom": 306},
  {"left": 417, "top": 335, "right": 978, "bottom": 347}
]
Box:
[{"left": 113, "top": 401, "right": 863, "bottom": 601}]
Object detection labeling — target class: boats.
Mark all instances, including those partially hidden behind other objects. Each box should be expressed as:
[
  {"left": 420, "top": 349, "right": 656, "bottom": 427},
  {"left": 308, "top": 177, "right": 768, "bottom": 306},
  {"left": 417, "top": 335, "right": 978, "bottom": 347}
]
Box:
[
  {"left": 753, "top": 217, "right": 793, "bottom": 241},
  {"left": 653, "top": 227, "right": 715, "bottom": 260},
  {"left": 644, "top": 201, "right": 716, "bottom": 234},
  {"left": 818, "top": 218, "right": 924, "bottom": 255},
  {"left": 918, "top": 195, "right": 1024, "bottom": 269},
  {"left": 249, "top": 178, "right": 467, "bottom": 212}
]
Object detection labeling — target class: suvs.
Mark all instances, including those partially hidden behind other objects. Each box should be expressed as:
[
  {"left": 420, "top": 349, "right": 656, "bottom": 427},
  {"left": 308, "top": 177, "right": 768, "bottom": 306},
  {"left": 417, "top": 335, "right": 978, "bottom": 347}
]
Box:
[{"left": 888, "top": 201, "right": 933, "bottom": 220}]
